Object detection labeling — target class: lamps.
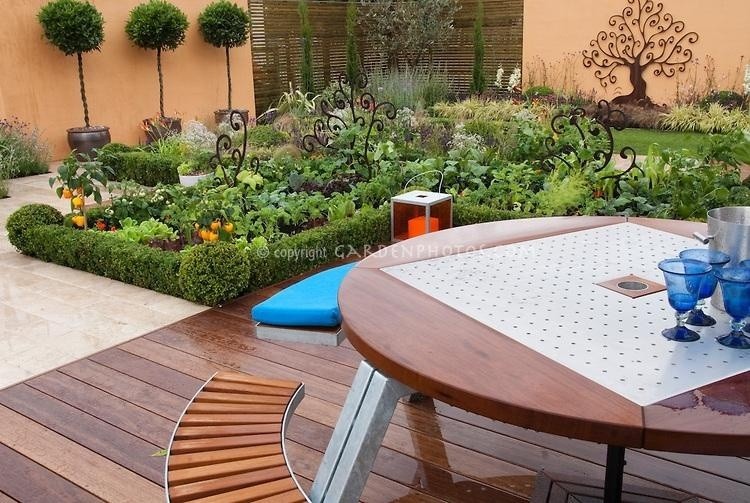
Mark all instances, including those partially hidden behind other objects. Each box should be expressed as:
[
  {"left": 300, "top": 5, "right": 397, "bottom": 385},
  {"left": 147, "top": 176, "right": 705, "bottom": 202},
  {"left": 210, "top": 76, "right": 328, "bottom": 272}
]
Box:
[{"left": 390, "top": 188, "right": 454, "bottom": 243}]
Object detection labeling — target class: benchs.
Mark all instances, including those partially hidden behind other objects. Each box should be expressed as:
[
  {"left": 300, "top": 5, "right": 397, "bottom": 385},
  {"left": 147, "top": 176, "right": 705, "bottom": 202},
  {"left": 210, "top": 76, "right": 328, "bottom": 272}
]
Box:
[
  {"left": 250, "top": 261, "right": 357, "bottom": 347},
  {"left": 164, "top": 370, "right": 313, "bottom": 503}
]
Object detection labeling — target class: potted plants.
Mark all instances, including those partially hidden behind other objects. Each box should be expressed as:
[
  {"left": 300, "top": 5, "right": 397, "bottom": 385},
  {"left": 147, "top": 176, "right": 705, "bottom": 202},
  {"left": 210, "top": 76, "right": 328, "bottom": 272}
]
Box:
[
  {"left": 196, "top": 0, "right": 252, "bottom": 126},
  {"left": 122, "top": 0, "right": 192, "bottom": 145},
  {"left": 36, "top": 0, "right": 111, "bottom": 162}
]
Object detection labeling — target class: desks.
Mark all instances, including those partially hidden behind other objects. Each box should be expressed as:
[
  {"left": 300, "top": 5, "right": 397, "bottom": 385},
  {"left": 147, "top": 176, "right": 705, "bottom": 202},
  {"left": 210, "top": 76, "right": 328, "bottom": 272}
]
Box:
[{"left": 308, "top": 215, "right": 750, "bottom": 502}]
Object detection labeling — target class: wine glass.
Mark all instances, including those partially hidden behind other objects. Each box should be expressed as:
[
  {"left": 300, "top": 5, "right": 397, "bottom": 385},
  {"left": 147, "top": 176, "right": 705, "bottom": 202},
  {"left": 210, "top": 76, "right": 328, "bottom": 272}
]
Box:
[{"left": 658, "top": 249, "right": 750, "bottom": 349}]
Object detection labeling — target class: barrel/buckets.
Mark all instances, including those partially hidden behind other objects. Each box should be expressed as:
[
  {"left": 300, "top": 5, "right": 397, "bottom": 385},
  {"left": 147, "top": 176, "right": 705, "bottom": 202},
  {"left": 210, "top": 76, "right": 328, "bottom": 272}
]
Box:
[{"left": 690, "top": 206, "right": 750, "bottom": 312}]
